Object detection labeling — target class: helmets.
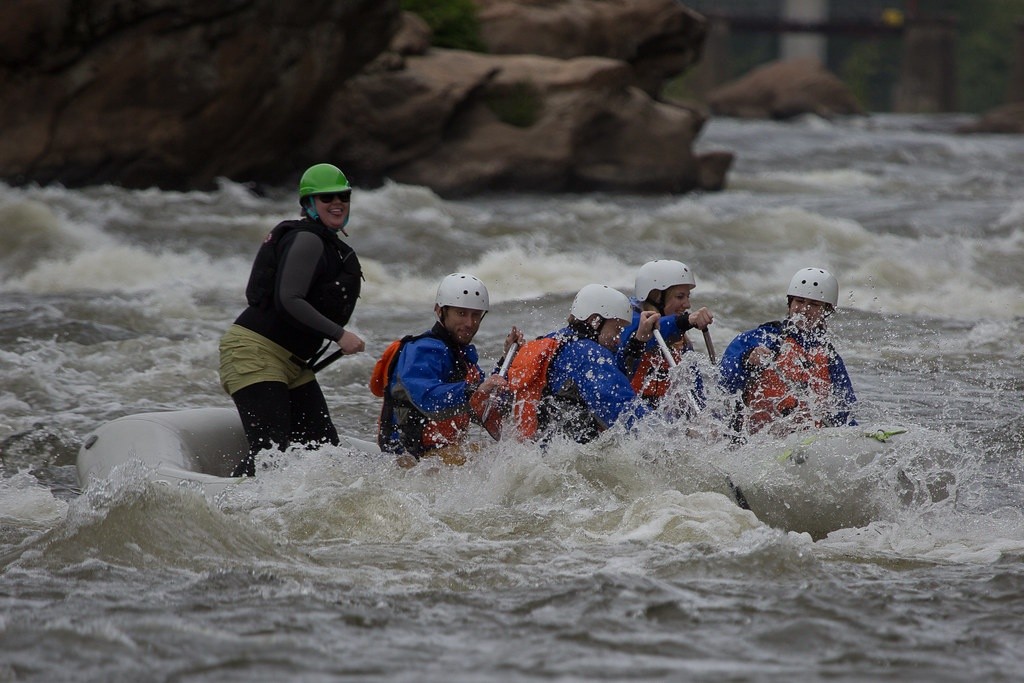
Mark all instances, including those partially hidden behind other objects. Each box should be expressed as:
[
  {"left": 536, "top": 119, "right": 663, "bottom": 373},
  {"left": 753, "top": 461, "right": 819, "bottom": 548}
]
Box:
[
  {"left": 569, "top": 284, "right": 633, "bottom": 328},
  {"left": 634, "top": 260, "right": 695, "bottom": 304},
  {"left": 297, "top": 164, "right": 352, "bottom": 205},
  {"left": 784, "top": 266, "right": 840, "bottom": 312},
  {"left": 434, "top": 272, "right": 492, "bottom": 317}
]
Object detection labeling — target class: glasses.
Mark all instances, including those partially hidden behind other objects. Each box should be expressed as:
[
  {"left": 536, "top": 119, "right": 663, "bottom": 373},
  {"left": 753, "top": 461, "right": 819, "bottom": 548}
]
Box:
[{"left": 316, "top": 191, "right": 351, "bottom": 204}]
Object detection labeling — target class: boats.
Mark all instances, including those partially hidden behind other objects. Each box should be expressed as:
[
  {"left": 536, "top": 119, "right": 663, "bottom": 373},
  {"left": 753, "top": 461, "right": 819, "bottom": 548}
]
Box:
[{"left": 72, "top": 400, "right": 957, "bottom": 525}]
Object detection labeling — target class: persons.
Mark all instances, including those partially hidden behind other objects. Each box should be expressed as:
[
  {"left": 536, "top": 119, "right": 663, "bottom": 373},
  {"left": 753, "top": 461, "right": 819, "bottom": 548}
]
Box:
[
  {"left": 718, "top": 268, "right": 859, "bottom": 445},
  {"left": 218, "top": 163, "right": 365, "bottom": 478},
  {"left": 377, "top": 273, "right": 526, "bottom": 462},
  {"left": 501, "top": 259, "right": 720, "bottom": 459}
]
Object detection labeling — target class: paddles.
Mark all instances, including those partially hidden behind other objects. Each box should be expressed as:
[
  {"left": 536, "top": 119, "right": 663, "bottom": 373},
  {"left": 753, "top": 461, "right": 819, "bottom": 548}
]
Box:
[
  {"left": 312, "top": 346, "right": 345, "bottom": 373},
  {"left": 760, "top": 356, "right": 834, "bottom": 429},
  {"left": 479, "top": 337, "right": 521, "bottom": 427},
  {"left": 695, "top": 321, "right": 717, "bottom": 365},
  {"left": 646, "top": 315, "right": 702, "bottom": 419}
]
